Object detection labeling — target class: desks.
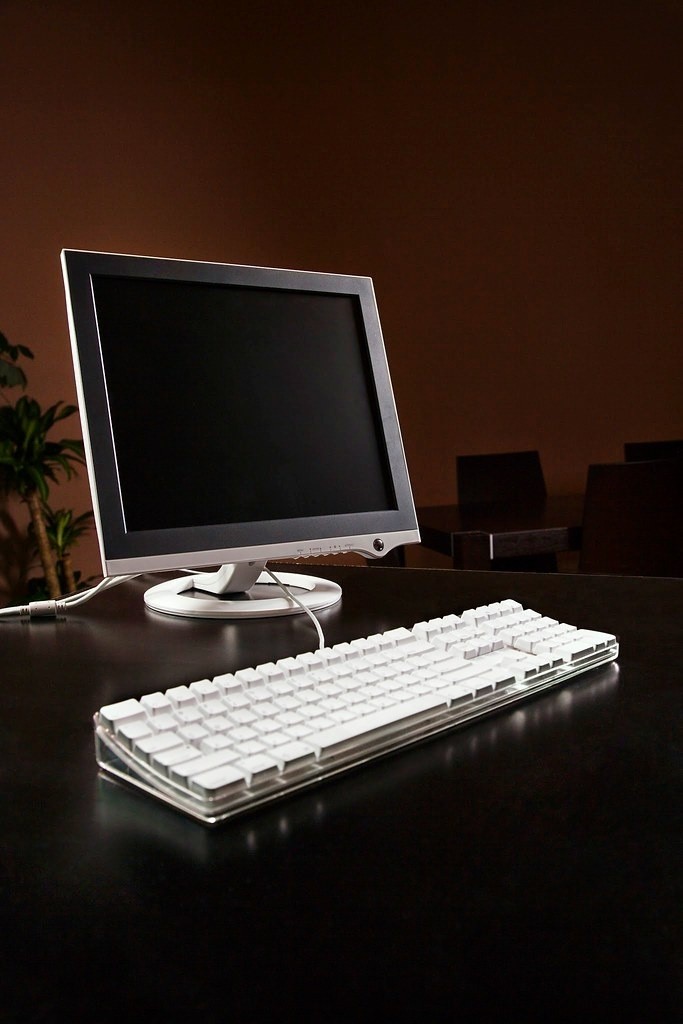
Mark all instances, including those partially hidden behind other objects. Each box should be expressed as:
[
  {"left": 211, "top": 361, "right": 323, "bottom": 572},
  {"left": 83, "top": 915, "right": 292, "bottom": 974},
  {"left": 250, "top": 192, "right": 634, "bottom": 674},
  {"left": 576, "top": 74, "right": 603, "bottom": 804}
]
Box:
[
  {"left": 375, "top": 492, "right": 584, "bottom": 571},
  {"left": 0, "top": 564, "right": 683, "bottom": 1024}
]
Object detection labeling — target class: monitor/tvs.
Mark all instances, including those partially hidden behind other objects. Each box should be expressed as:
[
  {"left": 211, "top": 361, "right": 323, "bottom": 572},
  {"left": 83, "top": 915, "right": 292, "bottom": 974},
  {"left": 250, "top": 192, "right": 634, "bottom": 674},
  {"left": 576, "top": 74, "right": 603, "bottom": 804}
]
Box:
[{"left": 58, "top": 248, "right": 421, "bottom": 619}]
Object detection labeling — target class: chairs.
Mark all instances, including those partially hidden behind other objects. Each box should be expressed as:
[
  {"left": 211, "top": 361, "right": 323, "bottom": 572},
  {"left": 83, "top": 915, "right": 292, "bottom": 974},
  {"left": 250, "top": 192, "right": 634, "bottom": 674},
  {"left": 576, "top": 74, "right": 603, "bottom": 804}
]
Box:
[
  {"left": 576, "top": 440, "right": 683, "bottom": 577},
  {"left": 456, "top": 451, "right": 557, "bottom": 573}
]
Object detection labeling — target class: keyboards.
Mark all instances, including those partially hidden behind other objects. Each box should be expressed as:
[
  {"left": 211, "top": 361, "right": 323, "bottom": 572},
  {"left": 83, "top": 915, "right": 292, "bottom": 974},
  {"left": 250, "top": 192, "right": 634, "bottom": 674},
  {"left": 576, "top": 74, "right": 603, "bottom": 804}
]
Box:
[{"left": 93, "top": 598, "right": 618, "bottom": 827}]
